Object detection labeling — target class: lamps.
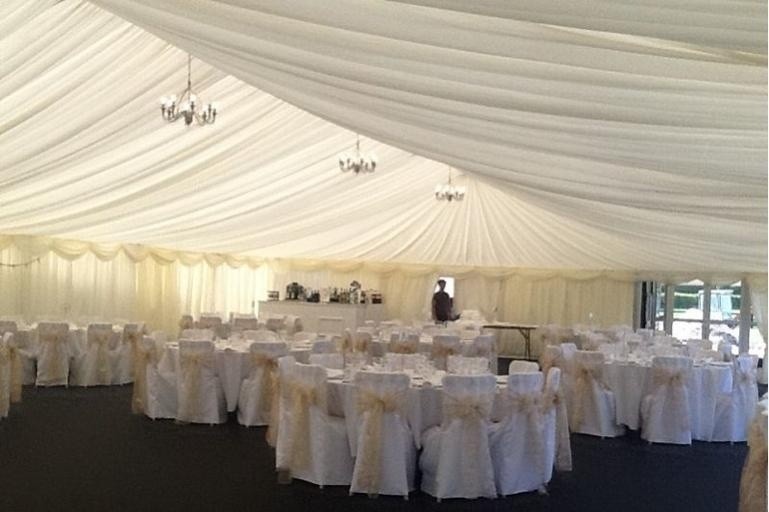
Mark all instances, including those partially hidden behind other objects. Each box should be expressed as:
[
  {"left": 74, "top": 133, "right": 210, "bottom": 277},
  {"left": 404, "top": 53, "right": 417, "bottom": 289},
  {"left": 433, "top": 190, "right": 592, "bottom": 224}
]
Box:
[
  {"left": 436, "top": 167, "right": 465, "bottom": 202},
  {"left": 159, "top": 54, "right": 218, "bottom": 126},
  {"left": 337, "top": 133, "right": 376, "bottom": 174}
]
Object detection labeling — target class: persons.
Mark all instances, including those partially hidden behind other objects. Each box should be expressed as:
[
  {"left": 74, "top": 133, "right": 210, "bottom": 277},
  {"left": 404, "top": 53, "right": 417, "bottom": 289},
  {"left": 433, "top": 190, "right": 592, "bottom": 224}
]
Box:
[{"left": 432, "top": 280, "right": 456, "bottom": 324}]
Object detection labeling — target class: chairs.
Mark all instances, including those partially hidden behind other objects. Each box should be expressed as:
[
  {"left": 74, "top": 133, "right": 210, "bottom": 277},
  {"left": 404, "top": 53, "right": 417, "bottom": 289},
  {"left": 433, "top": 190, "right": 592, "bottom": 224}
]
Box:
[
  {"left": 385, "top": 351, "right": 426, "bottom": 372},
  {"left": 719, "top": 363, "right": 756, "bottom": 445},
  {"left": 33, "top": 321, "right": 72, "bottom": 388},
  {"left": 571, "top": 349, "right": 624, "bottom": 439},
  {"left": 137, "top": 335, "right": 178, "bottom": 420},
  {"left": 641, "top": 356, "right": 694, "bottom": 445},
  {"left": 232, "top": 317, "right": 257, "bottom": 332},
  {"left": 266, "top": 317, "right": 285, "bottom": 334},
  {"left": 238, "top": 340, "right": 287, "bottom": 427},
  {"left": 390, "top": 332, "right": 420, "bottom": 351},
  {"left": 420, "top": 372, "right": 499, "bottom": 503},
  {"left": 561, "top": 340, "right": 576, "bottom": 358},
  {"left": 0, "top": 331, "right": 17, "bottom": 417},
  {"left": 685, "top": 338, "right": 714, "bottom": 348},
  {"left": 717, "top": 341, "right": 732, "bottom": 360},
  {"left": 241, "top": 330, "right": 277, "bottom": 343},
  {"left": 275, "top": 355, "right": 353, "bottom": 489},
  {"left": 353, "top": 328, "right": 372, "bottom": 353},
  {"left": 541, "top": 367, "right": 565, "bottom": 480},
  {"left": 494, "top": 372, "right": 548, "bottom": 498},
  {"left": 545, "top": 344, "right": 566, "bottom": 368},
  {"left": 2, "top": 320, "right": 19, "bottom": 332},
  {"left": 181, "top": 328, "right": 215, "bottom": 342},
  {"left": 431, "top": 335, "right": 461, "bottom": 360},
  {"left": 446, "top": 355, "right": 489, "bottom": 373},
  {"left": 199, "top": 315, "right": 221, "bottom": 332},
  {"left": 286, "top": 315, "right": 301, "bottom": 333},
  {"left": 348, "top": 371, "right": 414, "bottom": 501},
  {"left": 112, "top": 322, "right": 138, "bottom": 386},
  {"left": 309, "top": 355, "right": 346, "bottom": 368},
  {"left": 599, "top": 342, "right": 629, "bottom": 354},
  {"left": 72, "top": 322, "right": 112, "bottom": 388},
  {"left": 700, "top": 349, "right": 724, "bottom": 360},
  {"left": 174, "top": 339, "right": 225, "bottom": 427},
  {"left": 470, "top": 333, "right": 497, "bottom": 356},
  {"left": 508, "top": 361, "right": 539, "bottom": 374}
]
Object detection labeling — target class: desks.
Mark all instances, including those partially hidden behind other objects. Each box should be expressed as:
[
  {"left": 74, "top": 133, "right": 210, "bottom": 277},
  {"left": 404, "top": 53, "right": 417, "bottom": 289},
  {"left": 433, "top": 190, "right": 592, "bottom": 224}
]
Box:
[
  {"left": 257, "top": 298, "right": 383, "bottom": 335},
  {"left": 483, "top": 323, "right": 540, "bottom": 361}
]
